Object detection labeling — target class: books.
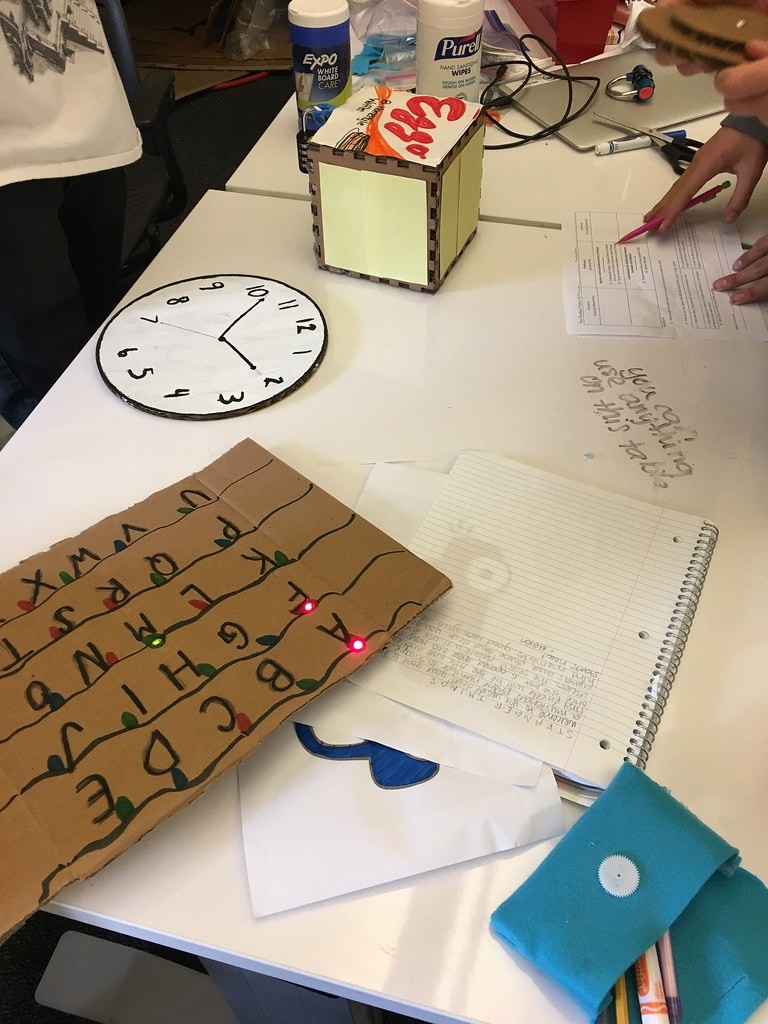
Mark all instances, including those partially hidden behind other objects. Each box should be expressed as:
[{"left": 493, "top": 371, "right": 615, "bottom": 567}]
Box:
[{"left": 344, "top": 450, "right": 719, "bottom": 810}]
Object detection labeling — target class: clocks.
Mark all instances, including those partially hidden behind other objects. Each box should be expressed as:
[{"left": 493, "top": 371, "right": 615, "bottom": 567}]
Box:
[{"left": 96, "top": 274, "right": 329, "bottom": 421}]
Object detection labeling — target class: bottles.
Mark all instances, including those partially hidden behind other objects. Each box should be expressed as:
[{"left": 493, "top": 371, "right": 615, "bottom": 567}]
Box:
[
  {"left": 285, "top": 0, "right": 356, "bottom": 131},
  {"left": 415, "top": 0, "right": 484, "bottom": 103}
]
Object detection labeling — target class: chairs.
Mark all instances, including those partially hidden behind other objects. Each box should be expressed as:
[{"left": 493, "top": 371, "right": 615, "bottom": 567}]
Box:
[{"left": 119, "top": 70, "right": 188, "bottom": 267}]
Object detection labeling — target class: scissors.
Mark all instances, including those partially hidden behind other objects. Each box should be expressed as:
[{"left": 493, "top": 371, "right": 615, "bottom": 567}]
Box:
[{"left": 593, "top": 111, "right": 704, "bottom": 175}]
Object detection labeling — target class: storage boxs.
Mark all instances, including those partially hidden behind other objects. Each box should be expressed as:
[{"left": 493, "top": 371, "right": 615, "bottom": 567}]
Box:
[{"left": 307, "top": 84, "right": 486, "bottom": 294}]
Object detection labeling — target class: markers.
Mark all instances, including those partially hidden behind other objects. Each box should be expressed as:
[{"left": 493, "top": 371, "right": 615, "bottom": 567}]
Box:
[{"left": 595, "top": 129, "right": 686, "bottom": 156}]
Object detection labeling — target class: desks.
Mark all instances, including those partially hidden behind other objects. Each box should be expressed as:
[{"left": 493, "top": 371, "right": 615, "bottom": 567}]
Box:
[
  {"left": 0, "top": 189, "right": 768, "bottom": 1024},
  {"left": 225, "top": 23, "right": 767, "bottom": 249}
]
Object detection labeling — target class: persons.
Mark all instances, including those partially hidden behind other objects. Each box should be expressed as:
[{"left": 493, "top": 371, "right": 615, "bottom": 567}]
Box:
[
  {"left": 1, "top": 2, "right": 141, "bottom": 434},
  {"left": 643, "top": 113, "right": 768, "bottom": 305},
  {"left": 654, "top": 1, "right": 768, "bottom": 126}
]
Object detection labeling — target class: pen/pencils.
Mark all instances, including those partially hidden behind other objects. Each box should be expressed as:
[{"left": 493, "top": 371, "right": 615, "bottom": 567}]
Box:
[
  {"left": 596, "top": 925, "right": 685, "bottom": 1024},
  {"left": 612, "top": 179, "right": 732, "bottom": 248}
]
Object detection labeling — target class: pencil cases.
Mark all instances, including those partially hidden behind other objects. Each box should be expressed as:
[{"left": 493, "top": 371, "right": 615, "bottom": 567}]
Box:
[{"left": 489, "top": 760, "right": 768, "bottom": 1024}]
